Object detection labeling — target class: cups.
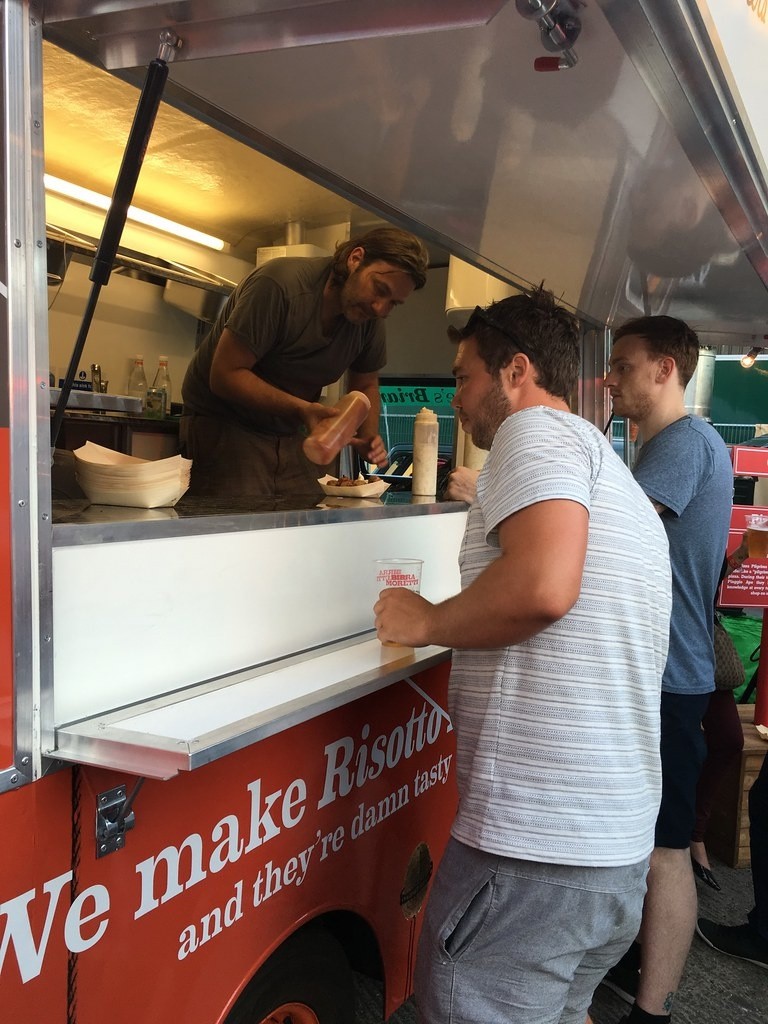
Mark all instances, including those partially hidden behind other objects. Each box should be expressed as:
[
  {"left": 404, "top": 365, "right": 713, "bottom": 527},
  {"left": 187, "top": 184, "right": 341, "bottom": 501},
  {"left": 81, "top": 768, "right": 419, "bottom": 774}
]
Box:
[{"left": 374, "top": 558, "right": 425, "bottom": 648}]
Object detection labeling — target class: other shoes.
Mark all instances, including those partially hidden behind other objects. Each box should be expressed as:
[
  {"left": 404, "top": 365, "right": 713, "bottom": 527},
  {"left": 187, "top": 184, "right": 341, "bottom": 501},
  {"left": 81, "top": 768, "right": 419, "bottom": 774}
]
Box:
[
  {"left": 692, "top": 854, "right": 721, "bottom": 891},
  {"left": 601, "top": 955, "right": 641, "bottom": 1004},
  {"left": 697, "top": 918, "right": 768, "bottom": 968}
]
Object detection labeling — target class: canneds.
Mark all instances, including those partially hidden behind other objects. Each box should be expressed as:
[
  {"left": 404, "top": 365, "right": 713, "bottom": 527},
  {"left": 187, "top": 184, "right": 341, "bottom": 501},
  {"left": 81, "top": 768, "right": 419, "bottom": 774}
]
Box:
[{"left": 146, "top": 387, "right": 167, "bottom": 419}]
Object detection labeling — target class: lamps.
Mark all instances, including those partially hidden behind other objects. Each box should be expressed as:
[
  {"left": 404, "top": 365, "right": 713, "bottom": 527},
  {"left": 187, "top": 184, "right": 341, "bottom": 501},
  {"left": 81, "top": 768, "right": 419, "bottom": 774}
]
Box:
[{"left": 42, "top": 173, "right": 234, "bottom": 254}]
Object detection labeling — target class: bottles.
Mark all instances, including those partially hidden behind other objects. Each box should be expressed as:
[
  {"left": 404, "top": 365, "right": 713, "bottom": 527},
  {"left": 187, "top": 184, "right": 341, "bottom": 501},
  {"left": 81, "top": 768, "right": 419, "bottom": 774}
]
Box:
[
  {"left": 127, "top": 354, "right": 147, "bottom": 416},
  {"left": 92, "top": 364, "right": 103, "bottom": 413},
  {"left": 303, "top": 390, "right": 372, "bottom": 465},
  {"left": 152, "top": 355, "right": 171, "bottom": 418},
  {"left": 412, "top": 406, "right": 438, "bottom": 497}
]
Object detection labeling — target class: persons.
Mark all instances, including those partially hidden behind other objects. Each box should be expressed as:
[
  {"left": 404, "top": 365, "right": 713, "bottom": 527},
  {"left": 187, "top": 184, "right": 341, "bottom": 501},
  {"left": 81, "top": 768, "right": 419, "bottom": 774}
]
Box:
[
  {"left": 181, "top": 221, "right": 428, "bottom": 491},
  {"left": 373, "top": 290, "right": 672, "bottom": 1024},
  {"left": 448, "top": 317, "right": 768, "bottom": 1024}
]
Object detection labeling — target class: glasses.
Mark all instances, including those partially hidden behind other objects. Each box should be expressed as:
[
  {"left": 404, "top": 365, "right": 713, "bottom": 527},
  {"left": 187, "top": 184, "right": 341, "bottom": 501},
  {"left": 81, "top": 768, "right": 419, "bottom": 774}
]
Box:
[{"left": 463, "top": 306, "right": 533, "bottom": 360}]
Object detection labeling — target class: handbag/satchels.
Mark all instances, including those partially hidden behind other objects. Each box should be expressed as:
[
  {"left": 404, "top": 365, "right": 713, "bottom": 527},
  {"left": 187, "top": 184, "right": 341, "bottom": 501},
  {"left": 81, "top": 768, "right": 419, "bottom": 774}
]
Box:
[{"left": 714, "top": 622, "right": 745, "bottom": 691}]
